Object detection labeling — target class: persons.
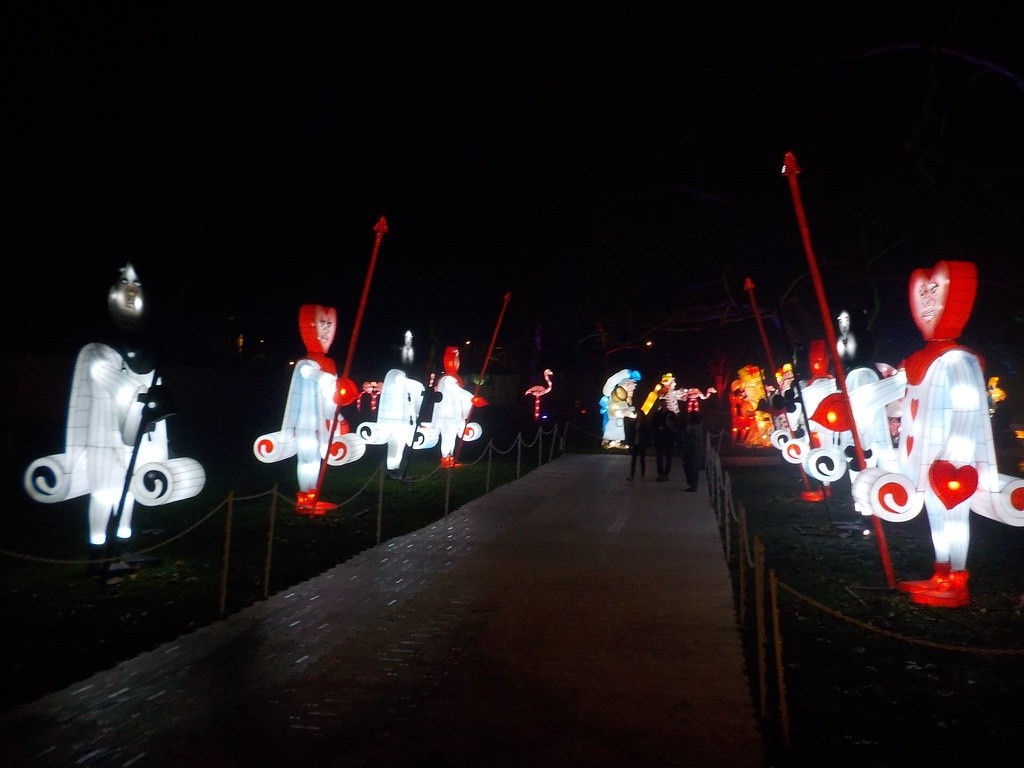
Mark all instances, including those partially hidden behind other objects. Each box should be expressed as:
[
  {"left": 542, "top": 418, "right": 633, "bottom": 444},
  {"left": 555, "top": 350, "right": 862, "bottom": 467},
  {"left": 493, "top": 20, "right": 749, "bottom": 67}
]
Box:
[
  {"left": 627, "top": 410, "right": 649, "bottom": 482},
  {"left": 649, "top": 398, "right": 703, "bottom": 493}
]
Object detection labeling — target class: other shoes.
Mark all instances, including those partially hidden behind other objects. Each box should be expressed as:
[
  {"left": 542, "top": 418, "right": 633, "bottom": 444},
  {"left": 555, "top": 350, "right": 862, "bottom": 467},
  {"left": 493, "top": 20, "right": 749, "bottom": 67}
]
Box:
[
  {"left": 639, "top": 475, "right": 644, "bottom": 483},
  {"left": 627, "top": 474, "right": 635, "bottom": 480},
  {"left": 656, "top": 474, "right": 662, "bottom": 480},
  {"left": 664, "top": 476, "right": 670, "bottom": 481},
  {"left": 686, "top": 487, "right": 698, "bottom": 492}
]
